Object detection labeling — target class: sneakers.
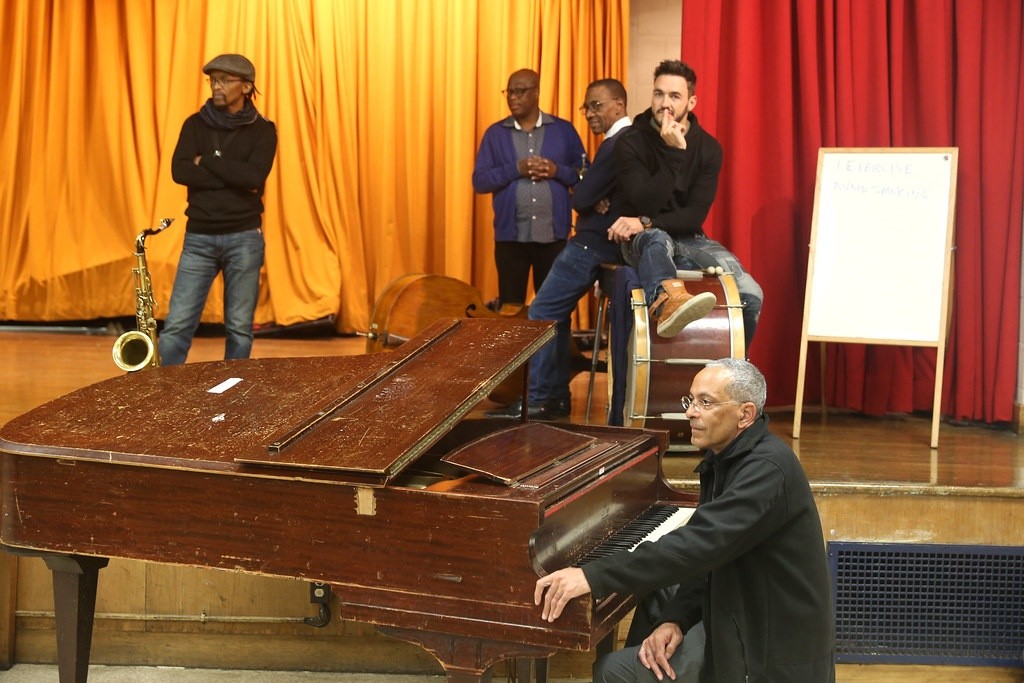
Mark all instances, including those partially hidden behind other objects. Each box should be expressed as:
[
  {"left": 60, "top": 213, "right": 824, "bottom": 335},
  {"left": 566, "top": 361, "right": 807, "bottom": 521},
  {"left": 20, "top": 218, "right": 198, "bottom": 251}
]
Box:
[{"left": 649, "top": 279, "right": 717, "bottom": 338}]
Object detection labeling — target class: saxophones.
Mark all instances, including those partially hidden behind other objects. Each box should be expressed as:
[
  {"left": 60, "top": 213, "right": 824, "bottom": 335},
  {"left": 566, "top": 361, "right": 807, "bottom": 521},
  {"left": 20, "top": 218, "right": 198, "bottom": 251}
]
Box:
[{"left": 109, "top": 216, "right": 175, "bottom": 374}]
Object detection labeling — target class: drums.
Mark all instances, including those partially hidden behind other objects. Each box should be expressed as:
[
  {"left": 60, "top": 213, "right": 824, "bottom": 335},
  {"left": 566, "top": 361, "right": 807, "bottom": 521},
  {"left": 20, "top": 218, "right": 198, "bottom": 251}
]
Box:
[{"left": 608, "top": 267, "right": 746, "bottom": 453}]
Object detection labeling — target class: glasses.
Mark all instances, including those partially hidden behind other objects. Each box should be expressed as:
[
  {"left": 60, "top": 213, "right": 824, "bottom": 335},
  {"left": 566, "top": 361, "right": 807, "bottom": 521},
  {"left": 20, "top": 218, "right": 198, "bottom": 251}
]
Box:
[
  {"left": 681, "top": 395, "right": 753, "bottom": 413},
  {"left": 207, "top": 76, "right": 243, "bottom": 86},
  {"left": 580, "top": 97, "right": 618, "bottom": 114},
  {"left": 501, "top": 87, "right": 537, "bottom": 99}
]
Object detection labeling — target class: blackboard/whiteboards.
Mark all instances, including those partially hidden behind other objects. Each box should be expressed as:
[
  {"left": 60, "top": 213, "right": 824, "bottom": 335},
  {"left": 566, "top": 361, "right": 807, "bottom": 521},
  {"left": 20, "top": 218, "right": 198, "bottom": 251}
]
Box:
[{"left": 799, "top": 146, "right": 958, "bottom": 346}]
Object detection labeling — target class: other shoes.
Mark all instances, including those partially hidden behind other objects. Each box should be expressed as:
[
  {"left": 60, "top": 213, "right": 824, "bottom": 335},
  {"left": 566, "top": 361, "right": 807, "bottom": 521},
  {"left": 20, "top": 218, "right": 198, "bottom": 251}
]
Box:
[
  {"left": 516, "top": 393, "right": 572, "bottom": 418},
  {"left": 483, "top": 399, "right": 549, "bottom": 421}
]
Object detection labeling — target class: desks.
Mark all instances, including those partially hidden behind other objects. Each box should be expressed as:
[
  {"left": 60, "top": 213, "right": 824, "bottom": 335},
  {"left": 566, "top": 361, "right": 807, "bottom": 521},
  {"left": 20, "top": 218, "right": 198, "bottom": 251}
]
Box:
[{"left": 585, "top": 264, "right": 626, "bottom": 422}]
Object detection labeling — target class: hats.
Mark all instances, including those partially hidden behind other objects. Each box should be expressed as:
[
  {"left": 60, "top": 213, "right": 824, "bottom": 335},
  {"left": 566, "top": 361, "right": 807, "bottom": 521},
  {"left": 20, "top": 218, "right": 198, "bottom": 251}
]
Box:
[{"left": 203, "top": 54, "right": 256, "bottom": 82}]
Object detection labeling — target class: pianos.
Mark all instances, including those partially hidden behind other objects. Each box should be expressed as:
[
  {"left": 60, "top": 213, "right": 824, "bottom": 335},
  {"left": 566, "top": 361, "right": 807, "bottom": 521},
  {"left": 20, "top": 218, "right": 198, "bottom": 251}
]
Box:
[{"left": 1, "top": 314, "right": 698, "bottom": 683}]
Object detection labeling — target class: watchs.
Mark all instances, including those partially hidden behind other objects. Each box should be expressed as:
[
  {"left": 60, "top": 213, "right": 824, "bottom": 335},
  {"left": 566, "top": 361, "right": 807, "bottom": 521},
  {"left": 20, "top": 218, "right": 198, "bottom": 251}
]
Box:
[{"left": 639, "top": 215, "right": 652, "bottom": 229}]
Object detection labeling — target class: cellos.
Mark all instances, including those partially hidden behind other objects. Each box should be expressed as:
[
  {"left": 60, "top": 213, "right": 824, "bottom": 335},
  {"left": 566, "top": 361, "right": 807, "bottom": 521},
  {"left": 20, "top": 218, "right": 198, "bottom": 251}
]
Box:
[{"left": 354, "top": 273, "right": 610, "bottom": 407}]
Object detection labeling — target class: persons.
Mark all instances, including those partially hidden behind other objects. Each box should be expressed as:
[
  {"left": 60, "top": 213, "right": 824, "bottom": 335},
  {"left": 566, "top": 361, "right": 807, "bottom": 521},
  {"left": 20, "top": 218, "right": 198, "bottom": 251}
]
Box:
[
  {"left": 470, "top": 59, "right": 765, "bottom": 428},
  {"left": 156, "top": 53, "right": 279, "bottom": 367},
  {"left": 533, "top": 358, "right": 837, "bottom": 683}
]
